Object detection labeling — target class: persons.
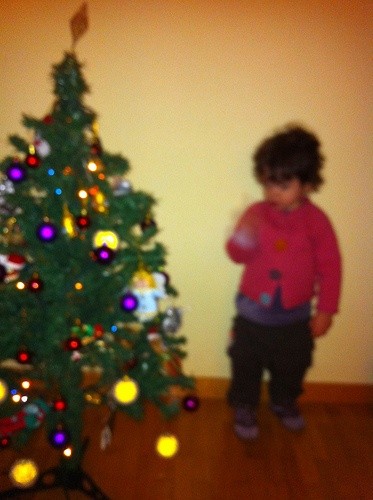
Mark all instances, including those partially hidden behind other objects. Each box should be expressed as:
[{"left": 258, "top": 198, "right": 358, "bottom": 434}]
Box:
[{"left": 224, "top": 125, "right": 344, "bottom": 438}]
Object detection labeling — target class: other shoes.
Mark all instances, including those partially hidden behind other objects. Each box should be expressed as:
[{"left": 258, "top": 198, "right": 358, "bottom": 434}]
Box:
[
  {"left": 271, "top": 403, "right": 306, "bottom": 433},
  {"left": 234, "top": 403, "right": 260, "bottom": 439}
]
